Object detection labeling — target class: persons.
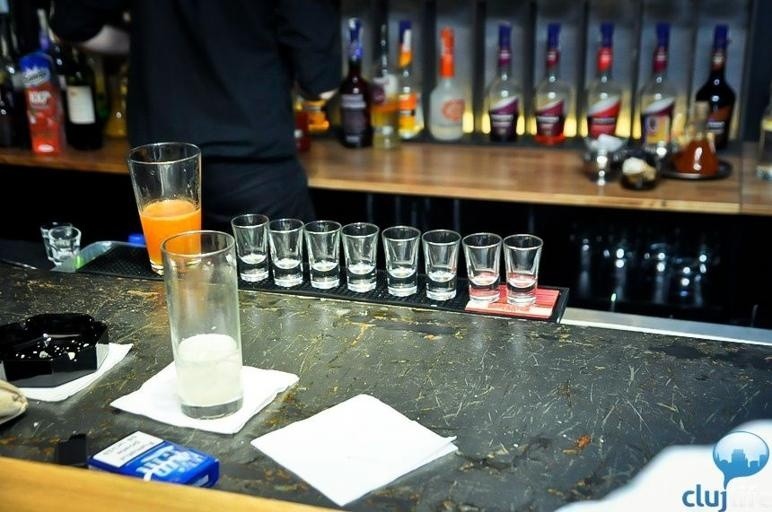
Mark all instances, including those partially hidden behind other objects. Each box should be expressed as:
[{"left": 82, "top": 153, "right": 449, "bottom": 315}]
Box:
[{"left": 50, "top": 1, "right": 342, "bottom": 262}]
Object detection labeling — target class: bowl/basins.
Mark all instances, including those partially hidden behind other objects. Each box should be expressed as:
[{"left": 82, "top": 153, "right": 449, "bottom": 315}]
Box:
[{"left": 581, "top": 151, "right": 624, "bottom": 187}]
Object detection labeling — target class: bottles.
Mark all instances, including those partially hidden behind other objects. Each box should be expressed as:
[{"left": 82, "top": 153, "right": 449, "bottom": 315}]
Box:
[
  {"left": 292, "top": 15, "right": 737, "bottom": 155},
  {"left": 752, "top": 85, "right": 772, "bottom": 180},
  {"left": 0, "top": 1, "right": 126, "bottom": 156}
]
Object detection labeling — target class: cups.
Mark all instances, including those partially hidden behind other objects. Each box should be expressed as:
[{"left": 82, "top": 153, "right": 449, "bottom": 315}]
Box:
[
  {"left": 40, "top": 219, "right": 82, "bottom": 267},
  {"left": 159, "top": 229, "right": 244, "bottom": 420},
  {"left": 231, "top": 213, "right": 543, "bottom": 308},
  {"left": 125, "top": 140, "right": 206, "bottom": 276}
]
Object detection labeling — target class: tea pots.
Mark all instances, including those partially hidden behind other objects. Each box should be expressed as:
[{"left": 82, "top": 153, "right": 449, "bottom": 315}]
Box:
[{"left": 672, "top": 119, "right": 722, "bottom": 176}]
